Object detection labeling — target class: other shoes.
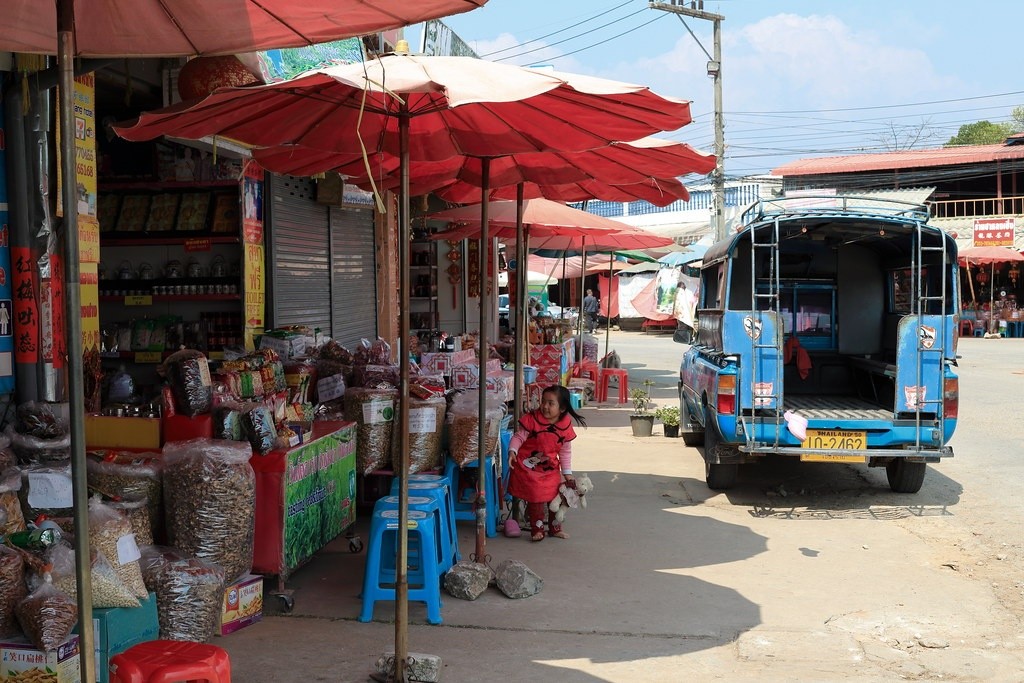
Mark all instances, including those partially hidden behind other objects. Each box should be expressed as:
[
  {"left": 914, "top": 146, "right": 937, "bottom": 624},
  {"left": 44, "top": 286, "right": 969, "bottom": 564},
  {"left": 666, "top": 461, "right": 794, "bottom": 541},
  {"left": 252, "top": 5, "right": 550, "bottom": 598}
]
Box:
[{"left": 504, "top": 519, "right": 522, "bottom": 538}]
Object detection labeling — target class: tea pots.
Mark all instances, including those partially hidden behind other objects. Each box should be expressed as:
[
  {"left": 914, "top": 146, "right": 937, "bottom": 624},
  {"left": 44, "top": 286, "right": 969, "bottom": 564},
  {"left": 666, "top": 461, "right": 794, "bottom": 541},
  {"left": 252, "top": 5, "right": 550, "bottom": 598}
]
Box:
[{"left": 98, "top": 254, "right": 241, "bottom": 279}]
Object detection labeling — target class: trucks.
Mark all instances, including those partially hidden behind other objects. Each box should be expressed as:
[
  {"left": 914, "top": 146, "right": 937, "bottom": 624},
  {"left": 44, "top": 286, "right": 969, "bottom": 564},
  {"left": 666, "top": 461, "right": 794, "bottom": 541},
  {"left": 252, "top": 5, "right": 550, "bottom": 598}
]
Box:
[{"left": 679, "top": 198, "right": 960, "bottom": 494}]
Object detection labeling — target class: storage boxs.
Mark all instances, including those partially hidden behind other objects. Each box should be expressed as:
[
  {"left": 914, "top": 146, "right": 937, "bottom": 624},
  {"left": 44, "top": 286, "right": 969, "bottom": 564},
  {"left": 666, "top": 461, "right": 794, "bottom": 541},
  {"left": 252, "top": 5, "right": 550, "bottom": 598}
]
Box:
[
  {"left": 960, "top": 310, "right": 976, "bottom": 320},
  {"left": 534, "top": 316, "right": 572, "bottom": 344},
  {"left": 1001, "top": 310, "right": 1024, "bottom": 322},
  {"left": 0, "top": 632, "right": 82, "bottom": 683},
  {"left": 998, "top": 320, "right": 1007, "bottom": 332},
  {"left": 214, "top": 574, "right": 264, "bottom": 637},
  {"left": 71, "top": 591, "right": 159, "bottom": 683},
  {"left": 84, "top": 412, "right": 160, "bottom": 449}
]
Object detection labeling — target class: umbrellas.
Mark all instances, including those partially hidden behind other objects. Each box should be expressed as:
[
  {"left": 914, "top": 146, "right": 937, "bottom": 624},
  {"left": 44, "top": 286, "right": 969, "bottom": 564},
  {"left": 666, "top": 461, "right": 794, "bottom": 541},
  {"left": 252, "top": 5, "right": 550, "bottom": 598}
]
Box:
[
  {"left": 104, "top": 35, "right": 698, "bottom": 683},
  {"left": 249, "top": 134, "right": 718, "bottom": 563},
  {"left": 414, "top": 176, "right": 709, "bottom": 439},
  {"left": 0, "top": 0, "right": 488, "bottom": 683},
  {"left": 958, "top": 246, "right": 1024, "bottom": 340}
]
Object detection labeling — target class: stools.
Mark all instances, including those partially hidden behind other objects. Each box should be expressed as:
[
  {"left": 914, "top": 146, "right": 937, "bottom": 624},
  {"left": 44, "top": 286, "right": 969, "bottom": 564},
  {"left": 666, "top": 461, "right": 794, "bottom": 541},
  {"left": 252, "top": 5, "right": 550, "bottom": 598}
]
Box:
[
  {"left": 390, "top": 474, "right": 462, "bottom": 562},
  {"left": 371, "top": 495, "right": 453, "bottom": 573},
  {"left": 573, "top": 364, "right": 600, "bottom": 401},
  {"left": 358, "top": 508, "right": 444, "bottom": 624},
  {"left": 998, "top": 330, "right": 1007, "bottom": 338},
  {"left": 1006, "top": 321, "right": 1018, "bottom": 337},
  {"left": 959, "top": 320, "right": 973, "bottom": 337},
  {"left": 390, "top": 482, "right": 456, "bottom": 573},
  {"left": 108, "top": 641, "right": 231, "bottom": 683},
  {"left": 974, "top": 328, "right": 984, "bottom": 337},
  {"left": 1019, "top": 321, "right": 1024, "bottom": 337},
  {"left": 598, "top": 367, "right": 628, "bottom": 404},
  {"left": 499, "top": 428, "right": 514, "bottom": 500},
  {"left": 445, "top": 455, "right": 501, "bottom": 538},
  {"left": 570, "top": 393, "right": 583, "bottom": 410}
]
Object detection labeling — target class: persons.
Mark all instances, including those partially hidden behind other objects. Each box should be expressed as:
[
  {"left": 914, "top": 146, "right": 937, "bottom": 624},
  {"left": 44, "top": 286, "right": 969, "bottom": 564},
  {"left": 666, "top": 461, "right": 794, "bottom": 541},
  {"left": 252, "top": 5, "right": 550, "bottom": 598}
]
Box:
[
  {"left": 508, "top": 384, "right": 588, "bottom": 541},
  {"left": 583, "top": 289, "right": 600, "bottom": 334}
]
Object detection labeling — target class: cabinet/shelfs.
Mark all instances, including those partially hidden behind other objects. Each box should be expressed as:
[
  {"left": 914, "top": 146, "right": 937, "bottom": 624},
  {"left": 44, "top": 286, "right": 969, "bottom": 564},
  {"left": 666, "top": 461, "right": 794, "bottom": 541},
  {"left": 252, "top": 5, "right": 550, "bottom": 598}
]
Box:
[
  {"left": 410, "top": 238, "right": 439, "bottom": 345},
  {"left": 420, "top": 348, "right": 515, "bottom": 402},
  {"left": 97, "top": 178, "right": 243, "bottom": 360}
]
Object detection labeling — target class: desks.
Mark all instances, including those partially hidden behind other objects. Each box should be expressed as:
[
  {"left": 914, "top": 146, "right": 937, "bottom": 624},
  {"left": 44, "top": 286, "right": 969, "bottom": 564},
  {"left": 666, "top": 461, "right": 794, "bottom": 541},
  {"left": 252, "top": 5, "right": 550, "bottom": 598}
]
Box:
[
  {"left": 216, "top": 420, "right": 364, "bottom": 613},
  {"left": 642, "top": 319, "right": 677, "bottom": 335},
  {"left": 491, "top": 338, "right": 575, "bottom": 403}
]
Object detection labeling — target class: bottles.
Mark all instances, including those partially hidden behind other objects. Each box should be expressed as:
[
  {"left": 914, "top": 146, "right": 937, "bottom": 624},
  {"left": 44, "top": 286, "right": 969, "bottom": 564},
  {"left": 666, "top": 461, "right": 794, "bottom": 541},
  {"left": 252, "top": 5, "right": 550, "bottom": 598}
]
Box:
[{"left": 200, "top": 311, "right": 244, "bottom": 352}]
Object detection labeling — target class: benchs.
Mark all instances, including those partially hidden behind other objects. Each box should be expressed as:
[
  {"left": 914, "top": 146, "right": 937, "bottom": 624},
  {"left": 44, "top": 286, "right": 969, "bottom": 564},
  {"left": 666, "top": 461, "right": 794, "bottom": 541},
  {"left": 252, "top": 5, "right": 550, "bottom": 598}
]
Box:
[{"left": 849, "top": 312, "right": 904, "bottom": 413}]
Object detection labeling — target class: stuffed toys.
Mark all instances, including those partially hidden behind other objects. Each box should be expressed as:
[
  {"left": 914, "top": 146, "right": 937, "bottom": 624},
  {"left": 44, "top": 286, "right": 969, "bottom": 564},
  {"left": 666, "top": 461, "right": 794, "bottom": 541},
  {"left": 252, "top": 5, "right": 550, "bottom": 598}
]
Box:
[{"left": 548, "top": 473, "right": 594, "bottom": 522}]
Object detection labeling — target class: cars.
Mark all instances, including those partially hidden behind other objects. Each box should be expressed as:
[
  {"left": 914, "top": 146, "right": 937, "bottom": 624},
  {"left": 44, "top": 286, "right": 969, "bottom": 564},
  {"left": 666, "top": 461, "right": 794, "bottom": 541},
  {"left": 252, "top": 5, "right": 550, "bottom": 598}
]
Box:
[{"left": 499, "top": 294, "right": 568, "bottom": 320}]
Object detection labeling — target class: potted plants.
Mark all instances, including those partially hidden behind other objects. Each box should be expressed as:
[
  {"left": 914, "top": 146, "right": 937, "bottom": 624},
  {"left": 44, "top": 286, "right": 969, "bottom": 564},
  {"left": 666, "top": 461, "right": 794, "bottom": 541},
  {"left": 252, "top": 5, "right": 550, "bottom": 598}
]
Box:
[
  {"left": 655, "top": 403, "right": 681, "bottom": 438},
  {"left": 627, "top": 379, "right": 654, "bottom": 437}
]
